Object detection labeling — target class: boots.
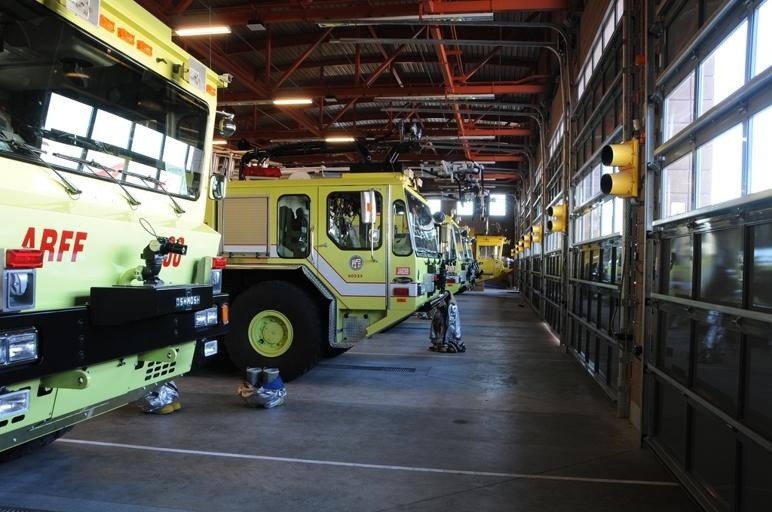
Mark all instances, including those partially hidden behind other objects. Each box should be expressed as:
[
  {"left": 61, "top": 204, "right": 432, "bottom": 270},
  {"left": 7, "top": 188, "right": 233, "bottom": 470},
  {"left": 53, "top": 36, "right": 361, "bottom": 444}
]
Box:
[{"left": 245, "top": 365, "right": 288, "bottom": 409}]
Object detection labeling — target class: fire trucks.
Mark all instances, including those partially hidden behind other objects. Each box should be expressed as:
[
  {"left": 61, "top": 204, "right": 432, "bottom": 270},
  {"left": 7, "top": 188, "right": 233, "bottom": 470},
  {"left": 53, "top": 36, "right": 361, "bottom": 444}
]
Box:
[{"left": 0, "top": 0, "right": 238, "bottom": 464}]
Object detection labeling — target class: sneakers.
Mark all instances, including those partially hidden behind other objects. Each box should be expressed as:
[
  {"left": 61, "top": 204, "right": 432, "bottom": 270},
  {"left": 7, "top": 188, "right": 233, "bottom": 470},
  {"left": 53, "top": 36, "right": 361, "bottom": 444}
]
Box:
[{"left": 154, "top": 400, "right": 183, "bottom": 415}]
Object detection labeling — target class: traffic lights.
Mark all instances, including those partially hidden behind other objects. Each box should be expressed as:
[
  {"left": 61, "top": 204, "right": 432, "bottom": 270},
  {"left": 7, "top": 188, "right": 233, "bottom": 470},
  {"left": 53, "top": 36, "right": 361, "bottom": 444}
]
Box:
[
  {"left": 601, "top": 139, "right": 638, "bottom": 197},
  {"left": 530, "top": 224, "right": 542, "bottom": 243},
  {"left": 513, "top": 235, "right": 530, "bottom": 256},
  {"left": 547, "top": 204, "right": 567, "bottom": 232}
]
